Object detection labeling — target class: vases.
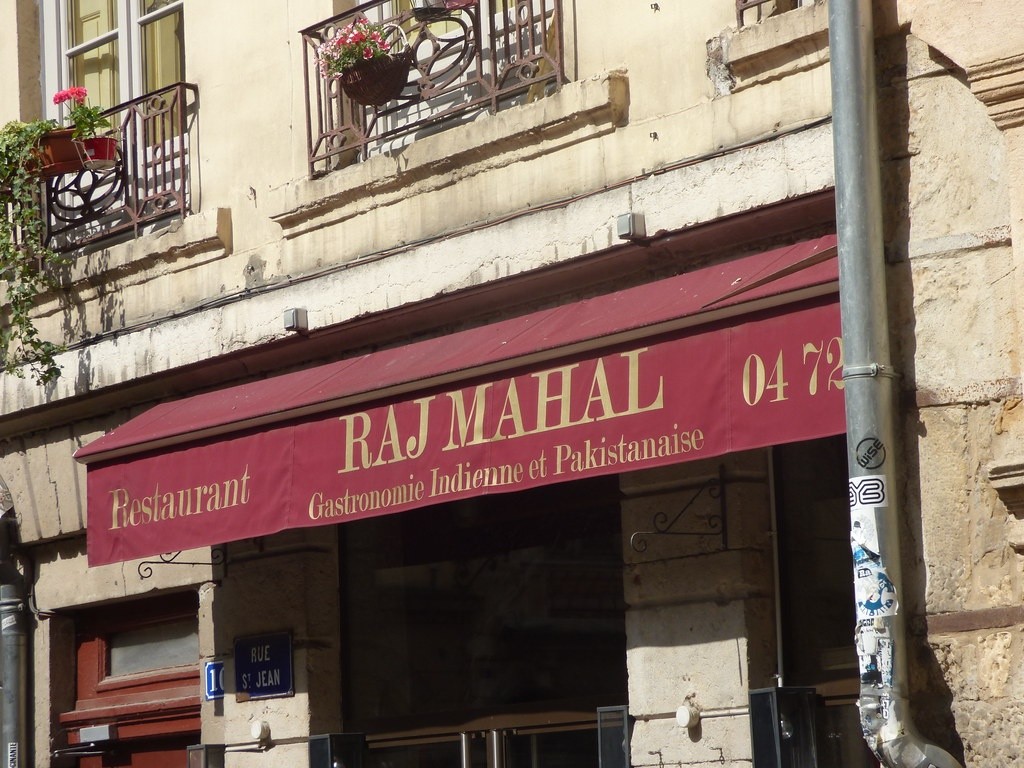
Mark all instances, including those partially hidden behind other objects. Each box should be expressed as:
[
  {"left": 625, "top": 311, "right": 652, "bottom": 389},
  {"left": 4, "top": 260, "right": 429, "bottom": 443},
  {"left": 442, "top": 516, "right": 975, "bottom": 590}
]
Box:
[{"left": 81, "top": 136, "right": 118, "bottom": 170}]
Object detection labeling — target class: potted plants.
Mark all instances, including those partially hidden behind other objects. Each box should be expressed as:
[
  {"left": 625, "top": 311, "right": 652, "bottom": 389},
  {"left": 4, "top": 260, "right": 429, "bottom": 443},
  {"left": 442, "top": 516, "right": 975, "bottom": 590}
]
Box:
[{"left": 0, "top": 119, "right": 80, "bottom": 388}]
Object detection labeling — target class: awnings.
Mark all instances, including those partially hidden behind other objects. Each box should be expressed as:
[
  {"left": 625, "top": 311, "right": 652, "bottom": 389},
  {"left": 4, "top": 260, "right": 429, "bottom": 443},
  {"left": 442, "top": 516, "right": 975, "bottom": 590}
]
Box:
[{"left": 75, "top": 219, "right": 847, "bottom": 569}]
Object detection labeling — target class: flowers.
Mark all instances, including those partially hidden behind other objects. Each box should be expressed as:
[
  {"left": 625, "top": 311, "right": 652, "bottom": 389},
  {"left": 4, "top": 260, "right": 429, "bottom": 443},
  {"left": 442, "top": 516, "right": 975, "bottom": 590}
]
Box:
[
  {"left": 312, "top": 17, "right": 390, "bottom": 78},
  {"left": 53, "top": 86, "right": 112, "bottom": 139}
]
